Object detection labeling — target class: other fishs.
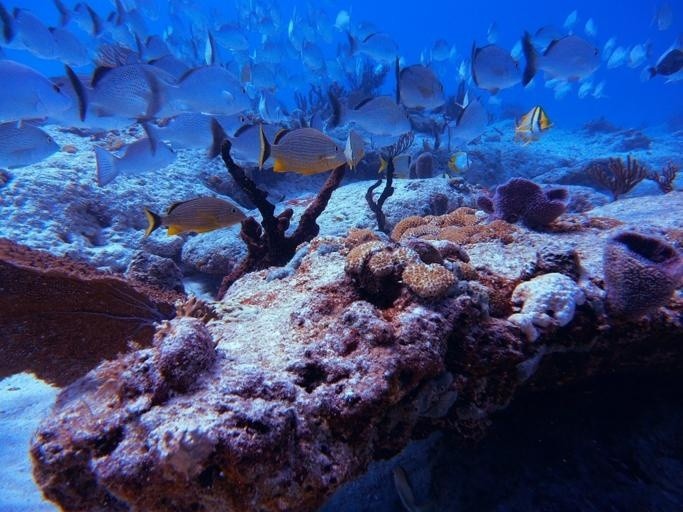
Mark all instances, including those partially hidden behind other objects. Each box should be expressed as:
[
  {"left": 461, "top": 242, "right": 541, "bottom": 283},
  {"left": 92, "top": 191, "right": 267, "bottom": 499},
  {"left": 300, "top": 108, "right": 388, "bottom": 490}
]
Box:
[{"left": 0, "top": 1, "right": 683, "bottom": 187}]
